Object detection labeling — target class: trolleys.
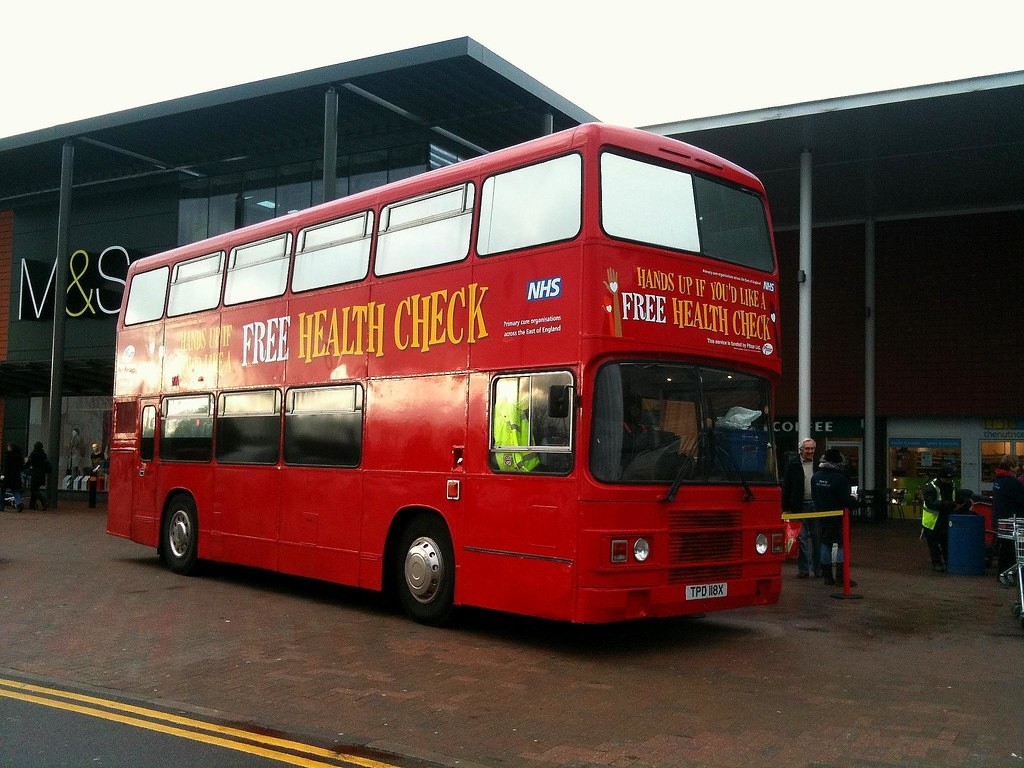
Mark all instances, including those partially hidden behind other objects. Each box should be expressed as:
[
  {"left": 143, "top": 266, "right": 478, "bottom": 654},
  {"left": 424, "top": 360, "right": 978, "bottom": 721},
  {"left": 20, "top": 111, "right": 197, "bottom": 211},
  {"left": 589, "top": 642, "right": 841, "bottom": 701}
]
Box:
[
  {"left": 10, "top": 468, "right": 31, "bottom": 508},
  {"left": 996, "top": 513, "right": 1023, "bottom": 627}
]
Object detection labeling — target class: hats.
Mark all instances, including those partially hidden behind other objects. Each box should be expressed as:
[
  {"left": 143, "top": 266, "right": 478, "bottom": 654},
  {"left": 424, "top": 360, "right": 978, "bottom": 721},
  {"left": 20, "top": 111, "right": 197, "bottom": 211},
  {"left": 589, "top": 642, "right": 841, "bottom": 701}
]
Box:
[
  {"left": 821, "top": 448, "right": 843, "bottom": 464},
  {"left": 939, "top": 465, "right": 954, "bottom": 478}
]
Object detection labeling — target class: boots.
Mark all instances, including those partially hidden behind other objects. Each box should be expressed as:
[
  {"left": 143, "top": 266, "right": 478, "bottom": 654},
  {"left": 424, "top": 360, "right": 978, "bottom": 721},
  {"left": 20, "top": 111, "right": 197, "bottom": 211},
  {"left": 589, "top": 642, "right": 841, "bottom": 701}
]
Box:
[
  {"left": 836, "top": 562, "right": 856, "bottom": 586},
  {"left": 822, "top": 564, "right": 836, "bottom": 585}
]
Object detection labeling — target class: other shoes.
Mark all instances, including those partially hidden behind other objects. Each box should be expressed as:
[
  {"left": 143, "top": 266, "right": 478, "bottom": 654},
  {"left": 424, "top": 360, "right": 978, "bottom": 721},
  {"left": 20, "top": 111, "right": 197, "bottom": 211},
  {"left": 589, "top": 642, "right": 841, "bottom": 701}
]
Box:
[
  {"left": 928, "top": 561, "right": 944, "bottom": 572},
  {"left": 17, "top": 503, "right": 24, "bottom": 513},
  {"left": 814, "top": 571, "right": 822, "bottom": 577},
  {"left": 0, "top": 508, "right": 4, "bottom": 511},
  {"left": 797, "top": 573, "right": 809, "bottom": 577}
]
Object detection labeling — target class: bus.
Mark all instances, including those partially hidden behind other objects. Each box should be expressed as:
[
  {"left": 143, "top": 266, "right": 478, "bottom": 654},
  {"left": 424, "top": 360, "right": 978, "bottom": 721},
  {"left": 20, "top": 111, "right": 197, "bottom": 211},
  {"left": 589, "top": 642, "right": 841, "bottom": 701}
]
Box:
[{"left": 104, "top": 123, "right": 785, "bottom": 625}]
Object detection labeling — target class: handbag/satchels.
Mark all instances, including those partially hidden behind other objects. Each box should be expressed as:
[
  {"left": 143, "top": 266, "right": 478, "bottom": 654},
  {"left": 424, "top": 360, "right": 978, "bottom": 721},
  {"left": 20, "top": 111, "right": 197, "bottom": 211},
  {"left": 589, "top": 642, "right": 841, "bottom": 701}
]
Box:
[{"left": 784, "top": 522, "right": 803, "bottom": 561}]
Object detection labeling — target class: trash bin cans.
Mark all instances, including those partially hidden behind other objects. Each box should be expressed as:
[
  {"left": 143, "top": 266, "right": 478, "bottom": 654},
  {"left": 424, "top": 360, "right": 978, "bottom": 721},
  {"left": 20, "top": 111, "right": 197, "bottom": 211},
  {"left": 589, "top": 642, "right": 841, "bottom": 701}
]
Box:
[{"left": 945, "top": 510, "right": 988, "bottom": 576}]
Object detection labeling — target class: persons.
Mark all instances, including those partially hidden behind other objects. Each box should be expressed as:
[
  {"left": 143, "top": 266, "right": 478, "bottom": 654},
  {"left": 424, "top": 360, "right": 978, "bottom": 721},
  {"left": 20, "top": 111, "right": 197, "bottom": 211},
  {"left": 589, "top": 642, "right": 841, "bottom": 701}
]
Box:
[
  {"left": 919, "top": 464, "right": 962, "bottom": 571},
  {"left": 782, "top": 437, "right": 858, "bottom": 587},
  {"left": 0, "top": 440, "right": 49, "bottom": 513},
  {"left": 991, "top": 455, "right": 1024, "bottom": 582},
  {"left": 493, "top": 384, "right": 549, "bottom": 473},
  {"left": 90, "top": 443, "right": 110, "bottom": 477},
  {"left": 65, "top": 428, "right": 86, "bottom": 476}
]
